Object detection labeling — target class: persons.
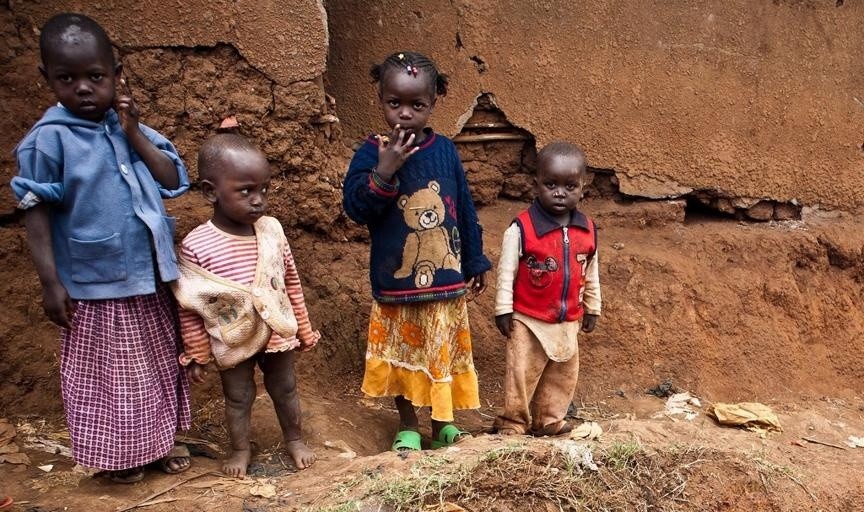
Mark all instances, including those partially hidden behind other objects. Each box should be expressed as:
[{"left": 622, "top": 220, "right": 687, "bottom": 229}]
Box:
[
  {"left": 170, "top": 129, "right": 324, "bottom": 482},
  {"left": 338, "top": 49, "right": 493, "bottom": 452},
  {"left": 493, "top": 139, "right": 605, "bottom": 442},
  {"left": 8, "top": 11, "right": 192, "bottom": 487}
]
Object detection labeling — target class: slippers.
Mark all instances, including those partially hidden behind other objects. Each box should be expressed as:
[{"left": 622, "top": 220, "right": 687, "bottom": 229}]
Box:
[
  {"left": 429, "top": 424, "right": 474, "bottom": 451},
  {"left": 108, "top": 466, "right": 145, "bottom": 485},
  {"left": 158, "top": 432, "right": 193, "bottom": 477},
  {"left": 390, "top": 426, "right": 422, "bottom": 457}
]
